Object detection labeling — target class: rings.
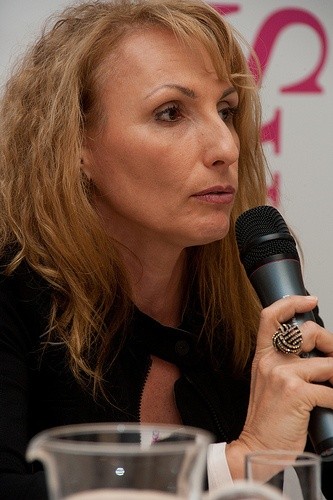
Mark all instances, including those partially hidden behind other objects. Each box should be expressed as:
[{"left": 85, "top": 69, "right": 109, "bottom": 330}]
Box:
[{"left": 271, "top": 321, "right": 304, "bottom": 358}]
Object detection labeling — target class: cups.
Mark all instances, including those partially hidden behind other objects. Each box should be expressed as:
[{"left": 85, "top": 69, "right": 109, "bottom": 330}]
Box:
[
  {"left": 25, "top": 422, "right": 214, "bottom": 500},
  {"left": 245, "top": 449, "right": 324, "bottom": 500}
]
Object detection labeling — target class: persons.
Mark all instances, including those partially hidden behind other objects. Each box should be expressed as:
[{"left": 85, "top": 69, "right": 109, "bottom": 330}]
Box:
[{"left": 0, "top": 0, "right": 332, "bottom": 500}]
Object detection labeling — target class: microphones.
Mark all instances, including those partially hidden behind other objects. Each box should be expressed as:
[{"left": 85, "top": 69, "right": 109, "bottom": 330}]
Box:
[{"left": 236, "top": 206, "right": 333, "bottom": 461}]
